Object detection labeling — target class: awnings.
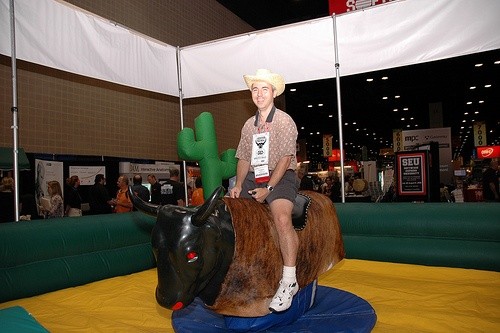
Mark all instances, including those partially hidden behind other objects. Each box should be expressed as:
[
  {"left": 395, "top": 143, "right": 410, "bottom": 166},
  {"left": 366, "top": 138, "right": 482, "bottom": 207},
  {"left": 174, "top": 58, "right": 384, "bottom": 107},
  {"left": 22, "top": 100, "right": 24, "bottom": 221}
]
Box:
[{"left": 0, "top": 146, "right": 32, "bottom": 171}]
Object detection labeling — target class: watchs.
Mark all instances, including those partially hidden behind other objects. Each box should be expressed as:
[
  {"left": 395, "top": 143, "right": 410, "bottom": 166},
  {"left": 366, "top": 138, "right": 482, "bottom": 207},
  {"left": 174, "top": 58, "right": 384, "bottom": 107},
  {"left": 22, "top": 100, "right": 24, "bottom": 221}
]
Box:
[{"left": 266, "top": 183, "right": 274, "bottom": 192}]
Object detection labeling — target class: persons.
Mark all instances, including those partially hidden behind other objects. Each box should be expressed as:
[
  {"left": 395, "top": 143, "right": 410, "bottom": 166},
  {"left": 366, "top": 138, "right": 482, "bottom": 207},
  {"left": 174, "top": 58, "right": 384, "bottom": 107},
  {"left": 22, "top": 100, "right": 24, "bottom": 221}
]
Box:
[
  {"left": 297, "top": 166, "right": 358, "bottom": 196},
  {"left": 0, "top": 171, "right": 14, "bottom": 218},
  {"left": 147, "top": 174, "right": 162, "bottom": 205},
  {"left": 192, "top": 177, "right": 206, "bottom": 207},
  {"left": 37, "top": 180, "right": 64, "bottom": 218},
  {"left": 128, "top": 174, "right": 150, "bottom": 212},
  {"left": 161, "top": 168, "right": 185, "bottom": 208},
  {"left": 229, "top": 65, "right": 304, "bottom": 313},
  {"left": 64, "top": 175, "right": 83, "bottom": 217},
  {"left": 87, "top": 174, "right": 113, "bottom": 214},
  {"left": 187, "top": 181, "right": 192, "bottom": 206},
  {"left": 106, "top": 176, "right": 134, "bottom": 213}
]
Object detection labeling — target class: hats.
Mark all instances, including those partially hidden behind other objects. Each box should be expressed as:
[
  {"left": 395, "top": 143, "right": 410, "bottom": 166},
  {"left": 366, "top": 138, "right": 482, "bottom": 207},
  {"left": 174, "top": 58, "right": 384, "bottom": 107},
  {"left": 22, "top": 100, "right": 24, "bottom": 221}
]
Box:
[{"left": 242, "top": 69, "right": 286, "bottom": 97}]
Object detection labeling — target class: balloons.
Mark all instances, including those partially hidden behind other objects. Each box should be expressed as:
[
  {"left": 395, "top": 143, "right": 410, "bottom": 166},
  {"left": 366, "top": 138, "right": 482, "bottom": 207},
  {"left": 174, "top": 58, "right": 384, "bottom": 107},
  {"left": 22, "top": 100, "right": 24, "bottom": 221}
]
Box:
[{"left": 176, "top": 111, "right": 237, "bottom": 202}]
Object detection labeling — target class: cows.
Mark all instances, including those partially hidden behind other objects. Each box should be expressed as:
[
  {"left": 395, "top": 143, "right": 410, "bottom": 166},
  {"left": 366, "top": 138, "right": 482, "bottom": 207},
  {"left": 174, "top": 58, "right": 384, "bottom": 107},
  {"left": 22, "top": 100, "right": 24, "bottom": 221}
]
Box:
[{"left": 127, "top": 184, "right": 346, "bottom": 318}]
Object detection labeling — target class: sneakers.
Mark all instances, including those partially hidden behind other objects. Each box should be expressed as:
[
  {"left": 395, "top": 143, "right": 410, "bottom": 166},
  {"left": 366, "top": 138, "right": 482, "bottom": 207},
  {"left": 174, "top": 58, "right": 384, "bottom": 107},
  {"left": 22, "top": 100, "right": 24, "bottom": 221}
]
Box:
[{"left": 269, "top": 278, "right": 299, "bottom": 313}]
